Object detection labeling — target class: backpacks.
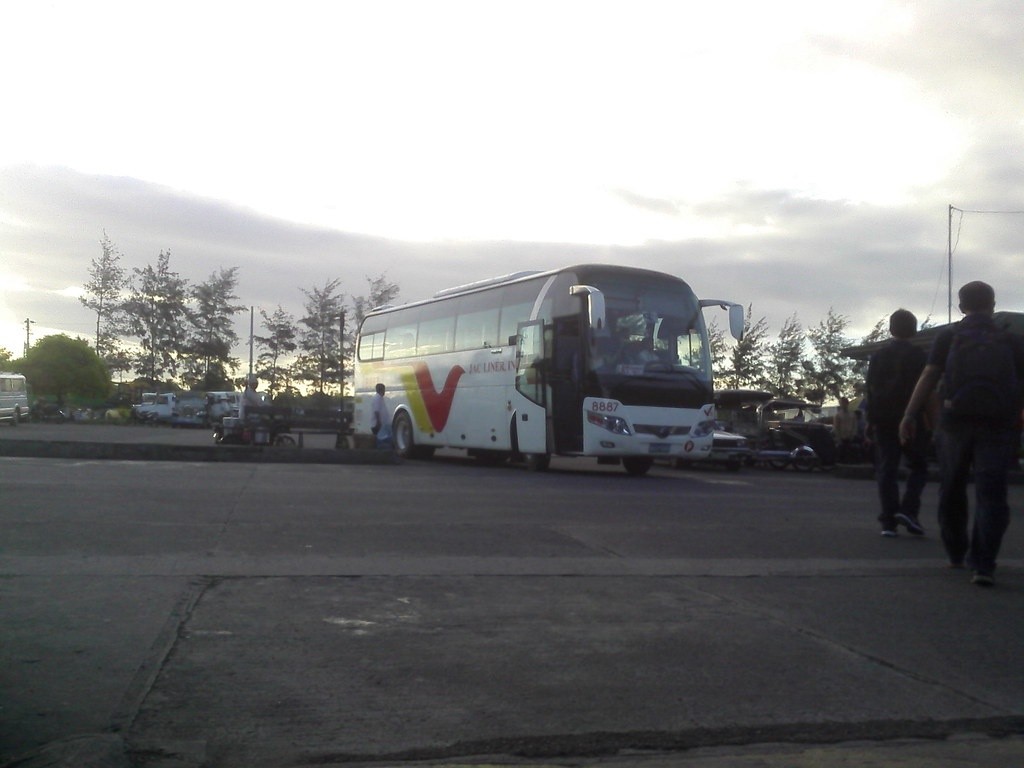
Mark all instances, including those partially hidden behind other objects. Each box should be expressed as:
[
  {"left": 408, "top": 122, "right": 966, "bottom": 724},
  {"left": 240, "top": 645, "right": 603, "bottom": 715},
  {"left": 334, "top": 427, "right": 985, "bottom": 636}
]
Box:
[{"left": 945, "top": 321, "right": 1022, "bottom": 419}]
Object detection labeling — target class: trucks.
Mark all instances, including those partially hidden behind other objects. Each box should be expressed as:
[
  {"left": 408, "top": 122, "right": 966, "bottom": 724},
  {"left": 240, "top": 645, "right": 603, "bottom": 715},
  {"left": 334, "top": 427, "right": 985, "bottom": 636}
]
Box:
[{"left": 131, "top": 391, "right": 273, "bottom": 426}]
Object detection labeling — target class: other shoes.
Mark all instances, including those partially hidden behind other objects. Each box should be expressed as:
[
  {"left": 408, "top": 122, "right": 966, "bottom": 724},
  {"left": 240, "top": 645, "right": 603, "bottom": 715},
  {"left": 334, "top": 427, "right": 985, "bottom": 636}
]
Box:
[
  {"left": 970, "top": 571, "right": 994, "bottom": 585},
  {"left": 881, "top": 527, "right": 896, "bottom": 537},
  {"left": 895, "top": 511, "right": 923, "bottom": 534},
  {"left": 949, "top": 558, "right": 965, "bottom": 568}
]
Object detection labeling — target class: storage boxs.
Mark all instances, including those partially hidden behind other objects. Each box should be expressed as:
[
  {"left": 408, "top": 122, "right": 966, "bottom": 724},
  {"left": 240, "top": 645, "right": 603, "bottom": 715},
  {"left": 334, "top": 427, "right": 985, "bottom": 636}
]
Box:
[{"left": 222, "top": 417, "right": 239, "bottom": 426}]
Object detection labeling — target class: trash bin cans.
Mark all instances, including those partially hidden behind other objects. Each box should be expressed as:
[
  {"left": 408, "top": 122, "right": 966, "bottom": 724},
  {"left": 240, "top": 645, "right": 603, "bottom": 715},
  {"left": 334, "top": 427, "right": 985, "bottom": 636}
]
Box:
[{"left": 222, "top": 415, "right": 240, "bottom": 443}]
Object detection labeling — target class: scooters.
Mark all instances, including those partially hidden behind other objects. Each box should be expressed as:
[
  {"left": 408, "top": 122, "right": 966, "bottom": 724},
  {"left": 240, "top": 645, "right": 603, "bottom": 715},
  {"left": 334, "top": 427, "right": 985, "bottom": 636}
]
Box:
[{"left": 212, "top": 395, "right": 297, "bottom": 447}]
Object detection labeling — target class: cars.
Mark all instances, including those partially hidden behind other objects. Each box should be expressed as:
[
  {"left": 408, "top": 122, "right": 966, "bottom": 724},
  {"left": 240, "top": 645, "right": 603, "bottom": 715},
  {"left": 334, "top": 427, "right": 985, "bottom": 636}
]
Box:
[{"left": 698, "top": 418, "right": 756, "bottom": 472}]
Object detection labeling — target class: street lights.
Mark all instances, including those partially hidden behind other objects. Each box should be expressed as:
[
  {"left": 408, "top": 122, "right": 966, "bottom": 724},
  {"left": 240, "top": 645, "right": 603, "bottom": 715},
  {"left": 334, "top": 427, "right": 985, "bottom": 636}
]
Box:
[{"left": 24, "top": 318, "right": 36, "bottom": 349}]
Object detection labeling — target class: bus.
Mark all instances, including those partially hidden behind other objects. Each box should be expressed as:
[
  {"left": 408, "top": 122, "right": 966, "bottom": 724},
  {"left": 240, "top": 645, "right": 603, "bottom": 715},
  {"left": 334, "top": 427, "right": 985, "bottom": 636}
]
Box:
[{"left": 352, "top": 261, "right": 745, "bottom": 475}]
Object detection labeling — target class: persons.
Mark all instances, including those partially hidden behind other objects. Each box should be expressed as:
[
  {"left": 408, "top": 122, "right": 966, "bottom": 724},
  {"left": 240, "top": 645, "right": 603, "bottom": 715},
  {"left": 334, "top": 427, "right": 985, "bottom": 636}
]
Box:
[
  {"left": 638, "top": 336, "right": 660, "bottom": 364},
  {"left": 833, "top": 397, "right": 858, "bottom": 445},
  {"left": 239, "top": 376, "right": 262, "bottom": 421},
  {"left": 898, "top": 281, "right": 1023, "bottom": 584},
  {"left": 861, "top": 310, "right": 939, "bottom": 539},
  {"left": 371, "top": 383, "right": 403, "bottom": 466}
]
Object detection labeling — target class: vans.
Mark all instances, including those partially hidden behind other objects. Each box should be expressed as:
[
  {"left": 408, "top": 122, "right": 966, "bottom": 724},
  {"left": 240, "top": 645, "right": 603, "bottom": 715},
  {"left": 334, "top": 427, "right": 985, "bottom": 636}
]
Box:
[{"left": 0, "top": 371, "right": 30, "bottom": 426}]
